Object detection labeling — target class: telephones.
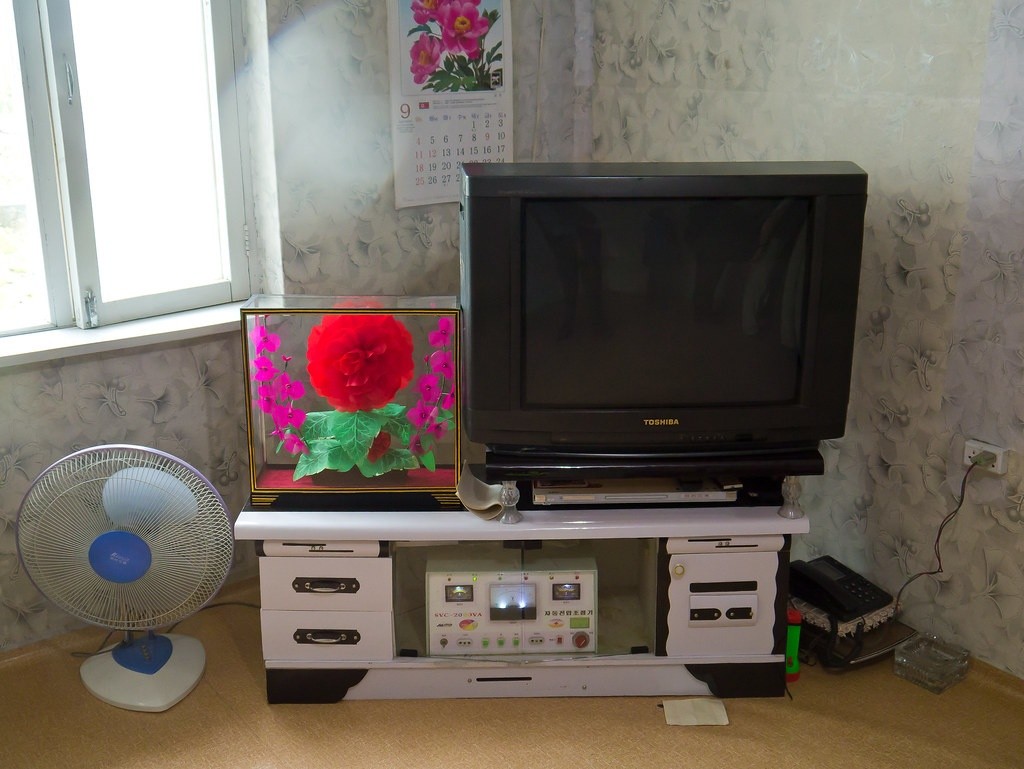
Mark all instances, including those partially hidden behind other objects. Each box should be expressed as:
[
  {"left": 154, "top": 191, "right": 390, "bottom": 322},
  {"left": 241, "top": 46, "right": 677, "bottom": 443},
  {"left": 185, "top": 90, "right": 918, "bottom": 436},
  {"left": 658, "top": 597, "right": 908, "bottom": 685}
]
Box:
[{"left": 789, "top": 554, "right": 900, "bottom": 640}]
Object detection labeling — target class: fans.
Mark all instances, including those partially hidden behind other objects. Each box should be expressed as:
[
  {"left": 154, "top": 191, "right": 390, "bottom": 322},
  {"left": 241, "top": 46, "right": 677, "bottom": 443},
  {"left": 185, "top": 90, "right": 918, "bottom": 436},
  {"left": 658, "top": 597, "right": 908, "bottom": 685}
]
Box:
[{"left": 14, "top": 442, "right": 236, "bottom": 714}]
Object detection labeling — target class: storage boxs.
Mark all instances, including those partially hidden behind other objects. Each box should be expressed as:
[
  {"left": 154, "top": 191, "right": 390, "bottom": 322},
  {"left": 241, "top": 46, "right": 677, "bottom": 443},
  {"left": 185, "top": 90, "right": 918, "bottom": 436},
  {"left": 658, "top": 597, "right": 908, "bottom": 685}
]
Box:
[{"left": 239, "top": 292, "right": 464, "bottom": 508}]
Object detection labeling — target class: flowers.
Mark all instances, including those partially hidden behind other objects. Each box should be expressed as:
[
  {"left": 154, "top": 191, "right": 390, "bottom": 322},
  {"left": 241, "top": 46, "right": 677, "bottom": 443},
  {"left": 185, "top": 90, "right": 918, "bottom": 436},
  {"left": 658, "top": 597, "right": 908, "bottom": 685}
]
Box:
[
  {"left": 405, "top": 1, "right": 504, "bottom": 93},
  {"left": 248, "top": 299, "right": 457, "bottom": 482}
]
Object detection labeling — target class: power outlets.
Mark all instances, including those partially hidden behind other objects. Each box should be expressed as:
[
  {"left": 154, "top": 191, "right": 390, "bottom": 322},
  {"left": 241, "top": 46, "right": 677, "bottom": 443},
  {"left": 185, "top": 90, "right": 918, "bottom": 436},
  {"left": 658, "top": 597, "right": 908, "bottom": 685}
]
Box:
[{"left": 963, "top": 439, "right": 1010, "bottom": 475}]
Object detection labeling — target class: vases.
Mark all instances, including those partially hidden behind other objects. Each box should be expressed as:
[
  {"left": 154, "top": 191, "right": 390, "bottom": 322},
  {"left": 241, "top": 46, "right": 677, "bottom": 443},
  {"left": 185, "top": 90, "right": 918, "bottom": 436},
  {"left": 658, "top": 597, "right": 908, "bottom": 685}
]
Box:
[{"left": 309, "top": 467, "right": 408, "bottom": 490}]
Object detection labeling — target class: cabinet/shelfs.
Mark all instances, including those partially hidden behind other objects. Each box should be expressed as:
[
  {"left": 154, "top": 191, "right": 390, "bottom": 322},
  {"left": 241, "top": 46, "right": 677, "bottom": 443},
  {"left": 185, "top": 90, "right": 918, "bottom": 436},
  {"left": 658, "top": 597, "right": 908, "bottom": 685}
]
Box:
[{"left": 232, "top": 464, "right": 810, "bottom": 708}]
